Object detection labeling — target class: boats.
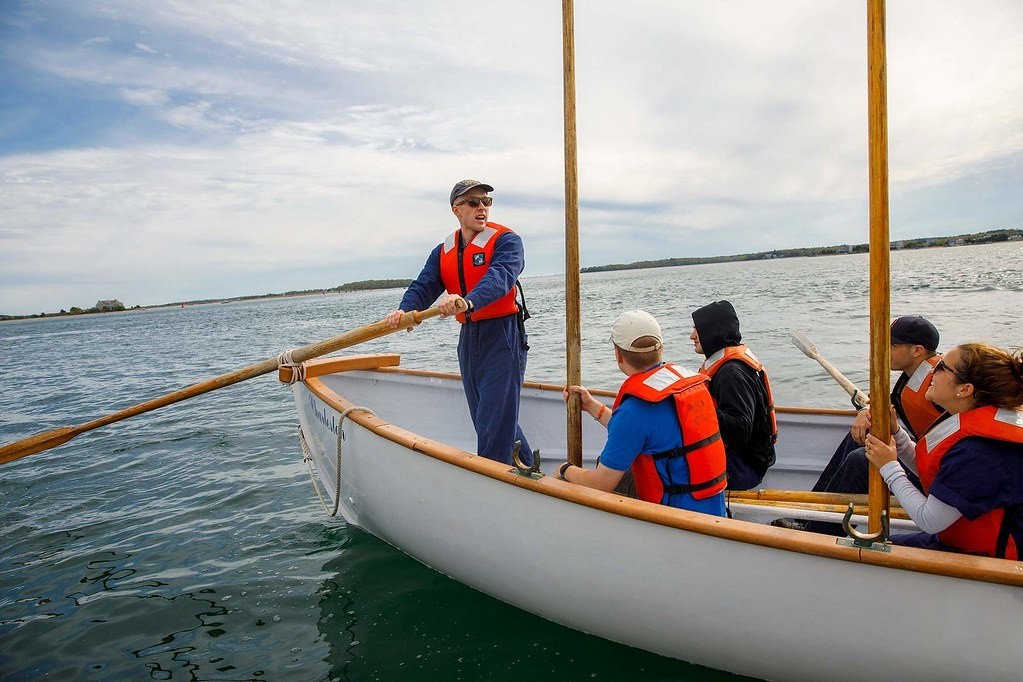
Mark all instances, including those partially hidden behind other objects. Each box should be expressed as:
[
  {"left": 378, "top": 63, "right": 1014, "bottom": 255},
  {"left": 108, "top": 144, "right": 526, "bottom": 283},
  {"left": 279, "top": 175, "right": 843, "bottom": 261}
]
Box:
[{"left": 279, "top": 0, "right": 1022, "bottom": 682}]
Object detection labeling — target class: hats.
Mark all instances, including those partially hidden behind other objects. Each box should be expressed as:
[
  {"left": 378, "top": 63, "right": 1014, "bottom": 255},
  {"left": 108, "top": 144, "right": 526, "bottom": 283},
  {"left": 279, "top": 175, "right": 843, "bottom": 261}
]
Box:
[
  {"left": 869, "top": 315, "right": 939, "bottom": 351},
  {"left": 606, "top": 310, "right": 663, "bottom": 352},
  {"left": 450, "top": 180, "right": 494, "bottom": 204}
]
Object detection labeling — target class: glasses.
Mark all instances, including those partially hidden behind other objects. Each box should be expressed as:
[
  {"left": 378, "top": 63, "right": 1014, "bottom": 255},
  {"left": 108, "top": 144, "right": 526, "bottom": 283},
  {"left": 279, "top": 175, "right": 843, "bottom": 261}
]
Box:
[
  {"left": 932, "top": 358, "right": 977, "bottom": 399},
  {"left": 456, "top": 196, "right": 492, "bottom": 207}
]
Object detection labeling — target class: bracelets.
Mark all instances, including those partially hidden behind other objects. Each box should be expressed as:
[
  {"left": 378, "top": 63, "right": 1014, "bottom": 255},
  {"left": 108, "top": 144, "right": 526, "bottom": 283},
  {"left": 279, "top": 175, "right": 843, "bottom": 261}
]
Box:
[
  {"left": 592, "top": 403, "right": 606, "bottom": 421},
  {"left": 560, "top": 461, "right": 574, "bottom": 481}
]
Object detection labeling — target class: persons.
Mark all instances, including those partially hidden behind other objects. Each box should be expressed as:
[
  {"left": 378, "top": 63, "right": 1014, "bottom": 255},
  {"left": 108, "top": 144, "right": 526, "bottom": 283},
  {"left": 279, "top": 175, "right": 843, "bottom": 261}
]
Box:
[
  {"left": 551, "top": 310, "right": 728, "bottom": 518},
  {"left": 689, "top": 299, "right": 777, "bottom": 491},
  {"left": 385, "top": 179, "right": 530, "bottom": 471},
  {"left": 770, "top": 313, "right": 1023, "bottom": 562}
]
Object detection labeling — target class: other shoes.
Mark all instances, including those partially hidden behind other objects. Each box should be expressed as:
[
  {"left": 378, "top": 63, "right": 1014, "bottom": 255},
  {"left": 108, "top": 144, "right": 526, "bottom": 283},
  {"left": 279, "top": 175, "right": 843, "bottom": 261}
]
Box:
[{"left": 767, "top": 517, "right": 807, "bottom": 530}]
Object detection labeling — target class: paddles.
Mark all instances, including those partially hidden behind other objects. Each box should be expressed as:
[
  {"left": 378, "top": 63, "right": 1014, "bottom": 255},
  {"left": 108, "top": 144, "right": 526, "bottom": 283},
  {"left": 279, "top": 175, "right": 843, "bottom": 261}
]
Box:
[
  {"left": 792, "top": 332, "right": 870, "bottom": 408},
  {"left": 0, "top": 299, "right": 469, "bottom": 463}
]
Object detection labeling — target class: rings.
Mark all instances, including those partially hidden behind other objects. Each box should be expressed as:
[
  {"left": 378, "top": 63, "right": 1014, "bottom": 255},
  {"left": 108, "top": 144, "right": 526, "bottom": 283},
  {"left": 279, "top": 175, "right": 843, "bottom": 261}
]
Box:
[{"left": 867, "top": 442, "right": 873, "bottom": 450}]
